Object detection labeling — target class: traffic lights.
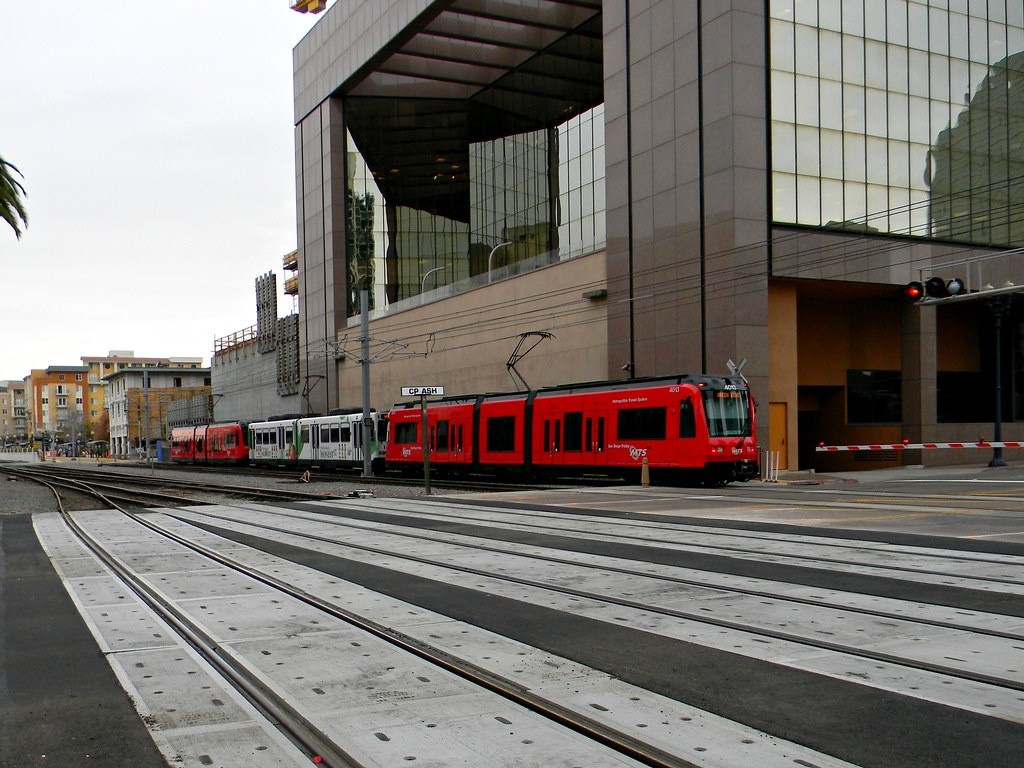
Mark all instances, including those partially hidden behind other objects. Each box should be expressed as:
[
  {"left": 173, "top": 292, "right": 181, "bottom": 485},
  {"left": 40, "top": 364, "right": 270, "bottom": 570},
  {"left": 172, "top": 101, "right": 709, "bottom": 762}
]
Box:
[
  {"left": 903, "top": 280, "right": 924, "bottom": 302},
  {"left": 926, "top": 277, "right": 964, "bottom": 300}
]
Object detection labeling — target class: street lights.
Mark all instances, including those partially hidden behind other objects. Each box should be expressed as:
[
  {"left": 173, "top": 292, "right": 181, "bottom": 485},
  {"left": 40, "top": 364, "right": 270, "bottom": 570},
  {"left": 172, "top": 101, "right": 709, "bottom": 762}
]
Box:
[
  {"left": 983, "top": 280, "right": 1018, "bottom": 469},
  {"left": 125, "top": 395, "right": 152, "bottom": 463}
]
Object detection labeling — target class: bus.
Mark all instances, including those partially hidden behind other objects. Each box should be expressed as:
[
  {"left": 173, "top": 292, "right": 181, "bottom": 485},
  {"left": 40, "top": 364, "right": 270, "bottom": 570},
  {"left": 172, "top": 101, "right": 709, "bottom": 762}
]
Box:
[
  {"left": 171, "top": 421, "right": 252, "bottom": 466},
  {"left": 383, "top": 331, "right": 763, "bottom": 490},
  {"left": 249, "top": 374, "right": 390, "bottom": 476},
  {"left": 88, "top": 440, "right": 110, "bottom": 452}
]
